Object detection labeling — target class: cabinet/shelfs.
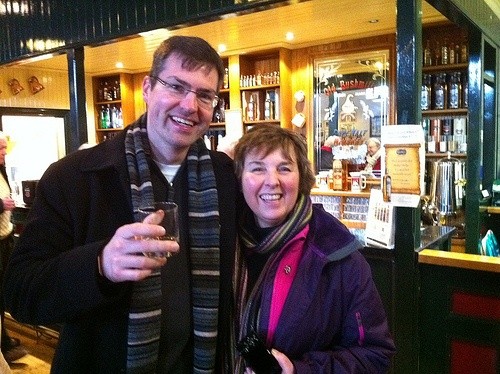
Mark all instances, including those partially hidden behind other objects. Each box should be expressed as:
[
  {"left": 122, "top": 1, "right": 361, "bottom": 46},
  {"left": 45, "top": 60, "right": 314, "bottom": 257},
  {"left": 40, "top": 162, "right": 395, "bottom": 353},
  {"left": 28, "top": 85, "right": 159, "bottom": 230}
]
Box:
[
  {"left": 202, "top": 47, "right": 292, "bottom": 136},
  {"left": 91, "top": 71, "right": 136, "bottom": 147},
  {"left": 421, "top": 19, "right": 468, "bottom": 159}
]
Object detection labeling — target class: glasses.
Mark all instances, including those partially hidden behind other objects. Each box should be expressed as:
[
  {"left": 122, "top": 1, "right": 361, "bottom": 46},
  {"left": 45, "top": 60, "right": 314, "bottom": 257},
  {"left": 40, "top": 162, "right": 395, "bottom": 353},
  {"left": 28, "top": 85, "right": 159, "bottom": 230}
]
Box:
[{"left": 150, "top": 73, "right": 221, "bottom": 111}]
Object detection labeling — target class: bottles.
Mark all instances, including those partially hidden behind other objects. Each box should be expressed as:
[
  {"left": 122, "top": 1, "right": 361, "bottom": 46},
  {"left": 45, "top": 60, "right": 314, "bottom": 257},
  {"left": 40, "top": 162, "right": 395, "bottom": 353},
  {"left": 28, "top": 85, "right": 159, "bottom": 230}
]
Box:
[
  {"left": 240, "top": 70, "right": 280, "bottom": 87},
  {"left": 100, "top": 104, "right": 123, "bottom": 128},
  {"left": 264, "top": 92, "right": 274, "bottom": 120},
  {"left": 200, "top": 126, "right": 225, "bottom": 152},
  {"left": 333, "top": 157, "right": 348, "bottom": 191},
  {"left": 424, "top": 34, "right": 469, "bottom": 66},
  {"left": 218, "top": 97, "right": 230, "bottom": 122},
  {"left": 223, "top": 67, "right": 229, "bottom": 89},
  {"left": 246, "top": 94, "right": 257, "bottom": 121},
  {"left": 421, "top": 72, "right": 468, "bottom": 111},
  {"left": 98, "top": 80, "right": 120, "bottom": 101}
]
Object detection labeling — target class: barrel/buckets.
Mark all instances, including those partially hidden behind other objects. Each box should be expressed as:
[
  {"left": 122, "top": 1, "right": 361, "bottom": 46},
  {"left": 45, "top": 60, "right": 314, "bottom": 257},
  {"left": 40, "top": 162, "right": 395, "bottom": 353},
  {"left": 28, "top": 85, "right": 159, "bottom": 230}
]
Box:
[{"left": 424, "top": 153, "right": 467, "bottom": 214}]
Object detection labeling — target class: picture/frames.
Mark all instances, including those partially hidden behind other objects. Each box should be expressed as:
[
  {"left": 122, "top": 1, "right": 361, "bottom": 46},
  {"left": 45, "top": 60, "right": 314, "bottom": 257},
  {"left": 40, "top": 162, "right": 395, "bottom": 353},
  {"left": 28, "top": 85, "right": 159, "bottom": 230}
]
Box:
[{"left": 305, "top": 43, "right": 396, "bottom": 187}]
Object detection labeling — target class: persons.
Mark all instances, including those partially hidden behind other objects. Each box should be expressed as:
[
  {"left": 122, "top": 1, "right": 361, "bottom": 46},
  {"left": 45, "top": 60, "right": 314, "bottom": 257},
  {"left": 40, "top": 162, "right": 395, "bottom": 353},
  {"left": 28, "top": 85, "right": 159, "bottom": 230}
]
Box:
[
  {"left": 231, "top": 125, "right": 396, "bottom": 374},
  {"left": 361, "top": 137, "right": 382, "bottom": 177},
  {"left": 0, "top": 132, "right": 30, "bottom": 360},
  {"left": 317, "top": 133, "right": 341, "bottom": 171},
  {"left": 4, "top": 33, "right": 262, "bottom": 374}
]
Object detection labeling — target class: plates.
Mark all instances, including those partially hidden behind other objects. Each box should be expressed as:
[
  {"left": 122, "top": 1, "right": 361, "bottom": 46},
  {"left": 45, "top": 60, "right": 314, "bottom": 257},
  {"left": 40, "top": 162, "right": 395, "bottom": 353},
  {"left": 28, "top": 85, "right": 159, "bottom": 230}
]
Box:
[{"left": 349, "top": 171, "right": 362, "bottom": 177}]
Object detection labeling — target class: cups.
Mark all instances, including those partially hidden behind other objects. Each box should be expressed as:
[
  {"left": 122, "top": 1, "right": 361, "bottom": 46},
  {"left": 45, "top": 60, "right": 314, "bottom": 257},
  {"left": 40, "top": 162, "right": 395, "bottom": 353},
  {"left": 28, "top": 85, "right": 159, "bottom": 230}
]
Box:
[
  {"left": 316, "top": 174, "right": 327, "bottom": 190},
  {"left": 448, "top": 135, "right": 457, "bottom": 152},
  {"left": 6, "top": 192, "right": 17, "bottom": 207},
  {"left": 458, "top": 134, "right": 467, "bottom": 153},
  {"left": 427, "top": 135, "right": 436, "bottom": 153},
  {"left": 137, "top": 201, "right": 180, "bottom": 258},
  {"left": 439, "top": 134, "right": 447, "bottom": 153},
  {"left": 326, "top": 174, "right": 334, "bottom": 189},
  {"left": 351, "top": 175, "right": 367, "bottom": 193}
]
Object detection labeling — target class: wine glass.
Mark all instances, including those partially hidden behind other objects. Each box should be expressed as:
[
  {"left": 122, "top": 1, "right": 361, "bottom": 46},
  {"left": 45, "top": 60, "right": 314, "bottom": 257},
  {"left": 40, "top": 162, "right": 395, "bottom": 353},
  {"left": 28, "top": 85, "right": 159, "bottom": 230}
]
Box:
[{"left": 215, "top": 110, "right": 223, "bottom": 123}]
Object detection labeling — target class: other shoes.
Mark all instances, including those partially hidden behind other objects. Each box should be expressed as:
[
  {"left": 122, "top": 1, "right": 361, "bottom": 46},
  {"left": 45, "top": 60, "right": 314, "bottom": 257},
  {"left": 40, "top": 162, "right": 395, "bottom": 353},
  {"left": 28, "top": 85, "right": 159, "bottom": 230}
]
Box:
[{"left": 7, "top": 337, "right": 22, "bottom": 346}]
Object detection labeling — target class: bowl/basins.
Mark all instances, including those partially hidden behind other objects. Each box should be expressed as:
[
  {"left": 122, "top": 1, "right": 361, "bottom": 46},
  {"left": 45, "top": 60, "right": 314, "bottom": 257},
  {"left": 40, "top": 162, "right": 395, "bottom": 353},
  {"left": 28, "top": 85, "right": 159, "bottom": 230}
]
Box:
[{"left": 319, "top": 171, "right": 329, "bottom": 174}]
